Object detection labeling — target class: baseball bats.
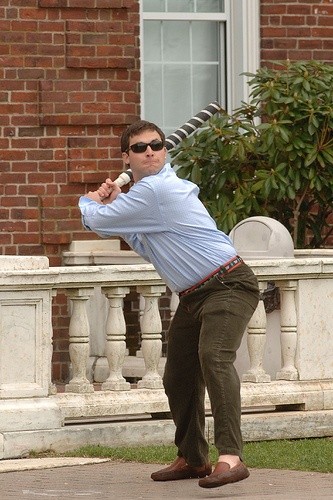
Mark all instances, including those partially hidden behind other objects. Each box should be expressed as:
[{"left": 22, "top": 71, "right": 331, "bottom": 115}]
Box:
[{"left": 94, "top": 101, "right": 222, "bottom": 205}]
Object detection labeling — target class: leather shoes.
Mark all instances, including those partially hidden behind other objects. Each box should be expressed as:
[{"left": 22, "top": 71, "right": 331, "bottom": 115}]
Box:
[
  {"left": 151, "top": 455, "right": 213, "bottom": 482},
  {"left": 198, "top": 460, "right": 250, "bottom": 488}
]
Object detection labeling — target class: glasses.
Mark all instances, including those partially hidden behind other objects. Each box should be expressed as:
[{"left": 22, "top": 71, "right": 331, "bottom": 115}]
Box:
[{"left": 125, "top": 138, "right": 166, "bottom": 154}]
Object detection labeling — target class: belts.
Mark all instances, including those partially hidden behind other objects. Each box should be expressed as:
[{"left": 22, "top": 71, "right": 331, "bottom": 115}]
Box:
[{"left": 178, "top": 256, "right": 244, "bottom": 295}]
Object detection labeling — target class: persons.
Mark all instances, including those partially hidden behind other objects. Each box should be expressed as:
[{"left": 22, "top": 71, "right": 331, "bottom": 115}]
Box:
[{"left": 78, "top": 120, "right": 260, "bottom": 489}]
